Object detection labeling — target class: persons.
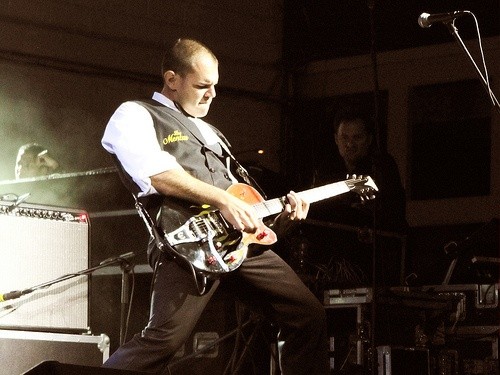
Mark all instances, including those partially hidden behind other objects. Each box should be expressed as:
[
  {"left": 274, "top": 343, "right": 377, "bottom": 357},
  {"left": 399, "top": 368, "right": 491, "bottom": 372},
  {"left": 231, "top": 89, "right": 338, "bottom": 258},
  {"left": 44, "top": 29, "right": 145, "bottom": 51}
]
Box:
[
  {"left": 15, "top": 146, "right": 84, "bottom": 177},
  {"left": 103, "top": 38, "right": 326, "bottom": 375},
  {"left": 310, "top": 105, "right": 410, "bottom": 289}
]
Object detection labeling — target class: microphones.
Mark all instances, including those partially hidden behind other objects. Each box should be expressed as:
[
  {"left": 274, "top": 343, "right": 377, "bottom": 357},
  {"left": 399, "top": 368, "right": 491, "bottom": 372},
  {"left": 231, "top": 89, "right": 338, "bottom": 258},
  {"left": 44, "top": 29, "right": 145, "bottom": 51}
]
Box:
[{"left": 418, "top": 10, "right": 469, "bottom": 28}]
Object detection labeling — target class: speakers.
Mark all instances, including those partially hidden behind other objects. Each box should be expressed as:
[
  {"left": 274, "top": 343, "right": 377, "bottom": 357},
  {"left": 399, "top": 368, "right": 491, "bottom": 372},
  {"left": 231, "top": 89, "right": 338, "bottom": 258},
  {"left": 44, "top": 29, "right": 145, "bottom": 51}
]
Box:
[
  {"left": 134, "top": 286, "right": 227, "bottom": 375},
  {"left": 0, "top": 199, "right": 91, "bottom": 331}
]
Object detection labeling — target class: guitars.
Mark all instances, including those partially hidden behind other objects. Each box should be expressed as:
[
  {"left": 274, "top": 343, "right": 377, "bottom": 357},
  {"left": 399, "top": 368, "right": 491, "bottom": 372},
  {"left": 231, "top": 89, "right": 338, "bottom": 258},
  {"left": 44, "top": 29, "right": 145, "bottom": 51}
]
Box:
[{"left": 155, "top": 174, "right": 380, "bottom": 280}]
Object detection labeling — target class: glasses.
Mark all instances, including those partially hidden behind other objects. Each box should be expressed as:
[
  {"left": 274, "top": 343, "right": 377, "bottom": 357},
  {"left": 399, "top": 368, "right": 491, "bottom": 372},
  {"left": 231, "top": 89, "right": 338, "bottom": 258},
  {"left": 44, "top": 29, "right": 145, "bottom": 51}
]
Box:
[{"left": 338, "top": 133, "right": 362, "bottom": 143}]
